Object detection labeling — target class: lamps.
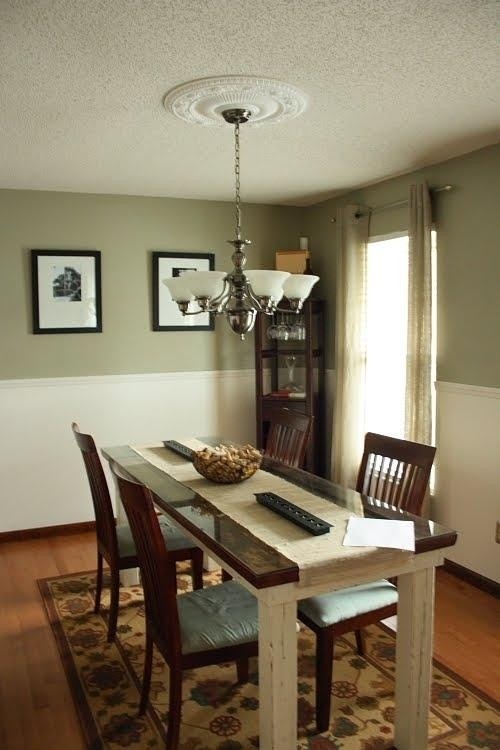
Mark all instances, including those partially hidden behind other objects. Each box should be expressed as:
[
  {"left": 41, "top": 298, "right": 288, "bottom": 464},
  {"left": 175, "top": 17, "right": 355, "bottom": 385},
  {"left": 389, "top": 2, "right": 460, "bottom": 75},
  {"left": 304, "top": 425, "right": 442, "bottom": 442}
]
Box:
[{"left": 162, "top": 77, "right": 320, "bottom": 342}]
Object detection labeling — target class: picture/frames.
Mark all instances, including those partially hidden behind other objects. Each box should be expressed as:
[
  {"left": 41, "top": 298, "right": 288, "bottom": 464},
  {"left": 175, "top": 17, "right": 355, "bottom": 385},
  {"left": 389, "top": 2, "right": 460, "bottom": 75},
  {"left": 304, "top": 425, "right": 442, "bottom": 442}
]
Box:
[{"left": 29, "top": 249, "right": 216, "bottom": 334}]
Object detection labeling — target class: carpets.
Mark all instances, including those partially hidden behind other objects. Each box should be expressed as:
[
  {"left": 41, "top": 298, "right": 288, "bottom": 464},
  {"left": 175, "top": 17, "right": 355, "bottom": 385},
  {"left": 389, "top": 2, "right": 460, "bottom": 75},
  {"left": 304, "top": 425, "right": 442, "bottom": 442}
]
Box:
[{"left": 35, "top": 558, "right": 500, "bottom": 750}]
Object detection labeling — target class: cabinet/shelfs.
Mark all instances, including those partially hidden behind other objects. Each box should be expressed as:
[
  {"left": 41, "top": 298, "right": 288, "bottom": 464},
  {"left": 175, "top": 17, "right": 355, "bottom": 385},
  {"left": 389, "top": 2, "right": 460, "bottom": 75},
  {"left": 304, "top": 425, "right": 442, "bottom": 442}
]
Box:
[{"left": 256, "top": 296, "right": 327, "bottom": 479}]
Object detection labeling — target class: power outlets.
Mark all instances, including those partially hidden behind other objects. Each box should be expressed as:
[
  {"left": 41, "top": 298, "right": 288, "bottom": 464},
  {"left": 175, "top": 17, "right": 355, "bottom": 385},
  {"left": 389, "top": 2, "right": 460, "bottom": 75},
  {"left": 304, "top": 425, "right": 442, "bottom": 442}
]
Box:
[{"left": 495, "top": 520, "right": 500, "bottom": 544}]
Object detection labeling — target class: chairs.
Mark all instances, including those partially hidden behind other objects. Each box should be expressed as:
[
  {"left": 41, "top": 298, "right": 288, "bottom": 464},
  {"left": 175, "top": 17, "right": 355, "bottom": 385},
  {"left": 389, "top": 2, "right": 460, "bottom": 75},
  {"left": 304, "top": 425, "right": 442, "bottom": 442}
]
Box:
[
  {"left": 73, "top": 424, "right": 204, "bottom": 646},
  {"left": 222, "top": 407, "right": 318, "bottom": 582},
  {"left": 108, "top": 460, "right": 301, "bottom": 747},
  {"left": 298, "top": 433, "right": 437, "bottom": 730}
]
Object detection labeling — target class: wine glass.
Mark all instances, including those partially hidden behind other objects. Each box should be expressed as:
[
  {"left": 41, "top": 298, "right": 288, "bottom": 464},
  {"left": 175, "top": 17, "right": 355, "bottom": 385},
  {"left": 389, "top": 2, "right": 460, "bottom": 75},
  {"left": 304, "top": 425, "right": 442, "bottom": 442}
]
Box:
[{"left": 268, "top": 311, "right": 306, "bottom": 342}]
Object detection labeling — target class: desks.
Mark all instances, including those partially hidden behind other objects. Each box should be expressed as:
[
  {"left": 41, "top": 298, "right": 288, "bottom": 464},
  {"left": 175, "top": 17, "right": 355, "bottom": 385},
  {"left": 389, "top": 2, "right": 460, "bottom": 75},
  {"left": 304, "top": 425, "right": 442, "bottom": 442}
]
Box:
[{"left": 99, "top": 438, "right": 457, "bottom": 750}]
